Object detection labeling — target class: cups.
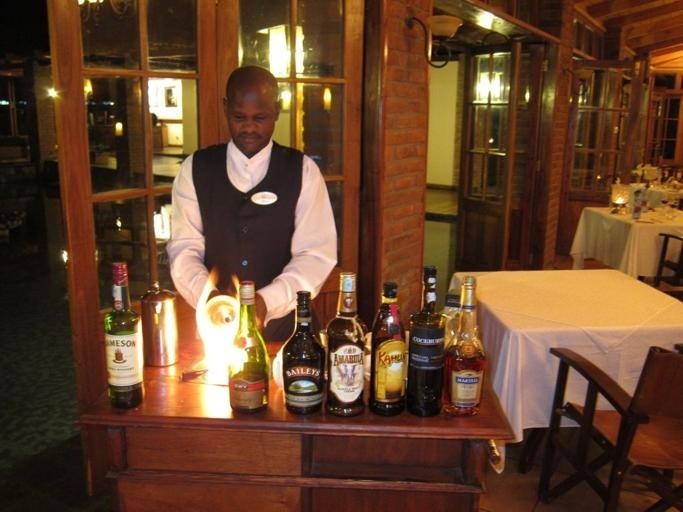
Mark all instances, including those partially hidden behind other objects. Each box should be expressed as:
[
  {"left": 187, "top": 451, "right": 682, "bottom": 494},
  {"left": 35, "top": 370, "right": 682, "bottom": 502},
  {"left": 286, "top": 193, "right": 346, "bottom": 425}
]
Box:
[{"left": 610, "top": 183, "right": 629, "bottom": 208}]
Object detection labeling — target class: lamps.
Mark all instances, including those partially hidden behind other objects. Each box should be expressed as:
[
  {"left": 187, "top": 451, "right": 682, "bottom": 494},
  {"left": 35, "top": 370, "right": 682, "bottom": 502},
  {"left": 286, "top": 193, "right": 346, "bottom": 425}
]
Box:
[{"left": 412, "top": 14, "right": 464, "bottom": 68}]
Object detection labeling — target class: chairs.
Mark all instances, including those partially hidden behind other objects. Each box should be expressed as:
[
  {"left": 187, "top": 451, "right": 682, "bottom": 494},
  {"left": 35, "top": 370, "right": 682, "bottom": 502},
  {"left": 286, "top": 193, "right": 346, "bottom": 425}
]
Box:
[
  {"left": 655, "top": 233, "right": 683, "bottom": 287},
  {"left": 539, "top": 343, "right": 683, "bottom": 512}
]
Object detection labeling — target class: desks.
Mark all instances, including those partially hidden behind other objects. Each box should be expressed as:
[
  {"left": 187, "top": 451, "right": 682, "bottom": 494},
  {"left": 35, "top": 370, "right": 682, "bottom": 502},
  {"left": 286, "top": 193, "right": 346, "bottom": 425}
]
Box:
[
  {"left": 569, "top": 207, "right": 683, "bottom": 282},
  {"left": 448, "top": 269, "right": 683, "bottom": 474},
  {"left": 80, "top": 340, "right": 515, "bottom": 512},
  {"left": 630, "top": 183, "right": 683, "bottom": 207}
]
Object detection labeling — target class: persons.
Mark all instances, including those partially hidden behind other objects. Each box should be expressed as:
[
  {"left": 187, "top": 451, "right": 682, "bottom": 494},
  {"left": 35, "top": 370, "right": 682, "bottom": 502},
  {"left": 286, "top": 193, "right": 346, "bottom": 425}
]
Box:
[{"left": 165, "top": 67, "right": 337, "bottom": 339}]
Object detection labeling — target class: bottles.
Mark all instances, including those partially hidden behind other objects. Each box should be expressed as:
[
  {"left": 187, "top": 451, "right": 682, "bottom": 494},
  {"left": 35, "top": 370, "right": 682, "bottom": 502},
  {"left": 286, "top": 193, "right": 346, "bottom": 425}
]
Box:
[
  {"left": 632, "top": 197, "right": 641, "bottom": 219},
  {"left": 140, "top": 279, "right": 181, "bottom": 369},
  {"left": 444, "top": 275, "right": 485, "bottom": 418},
  {"left": 323, "top": 271, "right": 366, "bottom": 417},
  {"left": 103, "top": 261, "right": 147, "bottom": 410},
  {"left": 407, "top": 263, "right": 443, "bottom": 417},
  {"left": 370, "top": 281, "right": 406, "bottom": 416},
  {"left": 225, "top": 279, "right": 271, "bottom": 413},
  {"left": 282, "top": 290, "right": 325, "bottom": 415}
]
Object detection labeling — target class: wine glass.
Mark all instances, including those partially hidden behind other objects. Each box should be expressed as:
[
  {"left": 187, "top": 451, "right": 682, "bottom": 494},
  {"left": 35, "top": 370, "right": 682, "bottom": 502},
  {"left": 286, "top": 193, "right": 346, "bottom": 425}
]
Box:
[{"left": 661, "top": 190, "right": 680, "bottom": 220}]
